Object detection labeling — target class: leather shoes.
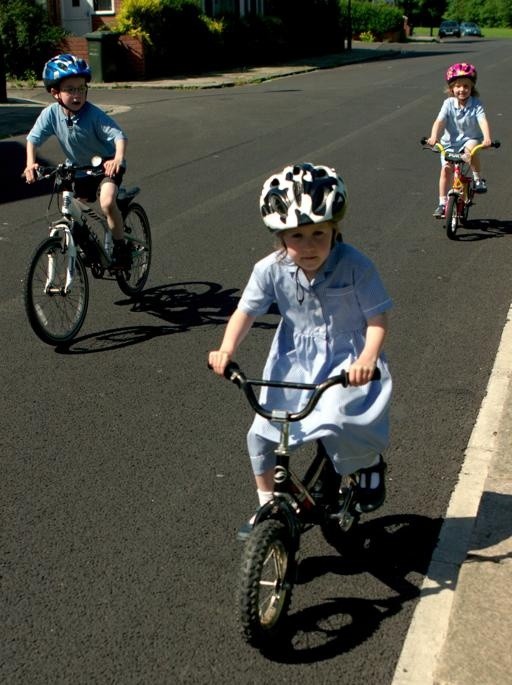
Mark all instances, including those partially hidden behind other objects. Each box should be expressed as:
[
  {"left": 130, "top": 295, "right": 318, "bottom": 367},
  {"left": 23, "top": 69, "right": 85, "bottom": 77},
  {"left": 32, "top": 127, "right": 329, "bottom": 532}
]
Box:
[
  {"left": 236, "top": 506, "right": 263, "bottom": 538},
  {"left": 356, "top": 453, "right": 387, "bottom": 512}
]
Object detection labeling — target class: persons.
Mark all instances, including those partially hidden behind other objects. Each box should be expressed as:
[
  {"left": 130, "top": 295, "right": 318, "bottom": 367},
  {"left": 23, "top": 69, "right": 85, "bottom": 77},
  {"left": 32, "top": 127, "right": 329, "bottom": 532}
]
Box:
[
  {"left": 424, "top": 61, "right": 493, "bottom": 220},
  {"left": 24, "top": 56, "right": 129, "bottom": 265},
  {"left": 208, "top": 162, "right": 395, "bottom": 542}
]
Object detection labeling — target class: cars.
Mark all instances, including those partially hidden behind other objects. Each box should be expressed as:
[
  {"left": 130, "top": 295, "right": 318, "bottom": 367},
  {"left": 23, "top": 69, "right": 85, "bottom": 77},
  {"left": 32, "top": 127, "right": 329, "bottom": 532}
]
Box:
[
  {"left": 438, "top": 19, "right": 461, "bottom": 39},
  {"left": 459, "top": 20, "right": 481, "bottom": 37}
]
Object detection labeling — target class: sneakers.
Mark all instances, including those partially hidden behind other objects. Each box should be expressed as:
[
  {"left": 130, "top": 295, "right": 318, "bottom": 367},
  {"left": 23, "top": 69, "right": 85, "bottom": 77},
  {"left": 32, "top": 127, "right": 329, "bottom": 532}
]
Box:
[
  {"left": 432, "top": 205, "right": 445, "bottom": 217},
  {"left": 113, "top": 246, "right": 131, "bottom": 268},
  {"left": 472, "top": 179, "right": 487, "bottom": 192}
]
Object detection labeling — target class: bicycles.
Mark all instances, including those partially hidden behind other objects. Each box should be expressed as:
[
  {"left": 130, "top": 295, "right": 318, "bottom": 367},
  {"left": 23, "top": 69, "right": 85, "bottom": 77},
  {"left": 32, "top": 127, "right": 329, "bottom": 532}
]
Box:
[
  {"left": 419, "top": 130, "right": 501, "bottom": 240},
  {"left": 199, "top": 349, "right": 390, "bottom": 649},
  {"left": 23, "top": 150, "right": 153, "bottom": 350}
]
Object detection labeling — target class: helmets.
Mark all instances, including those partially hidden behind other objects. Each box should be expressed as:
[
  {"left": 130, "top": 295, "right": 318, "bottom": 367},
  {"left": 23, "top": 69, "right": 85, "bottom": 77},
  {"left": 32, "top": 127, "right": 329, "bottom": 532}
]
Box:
[
  {"left": 259, "top": 163, "right": 347, "bottom": 231},
  {"left": 43, "top": 54, "right": 92, "bottom": 93},
  {"left": 446, "top": 63, "right": 476, "bottom": 84}
]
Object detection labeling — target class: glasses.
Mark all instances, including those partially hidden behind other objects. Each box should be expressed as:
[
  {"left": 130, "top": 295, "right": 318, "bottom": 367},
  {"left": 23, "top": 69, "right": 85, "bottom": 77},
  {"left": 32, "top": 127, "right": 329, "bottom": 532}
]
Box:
[{"left": 60, "top": 86, "right": 88, "bottom": 93}]
